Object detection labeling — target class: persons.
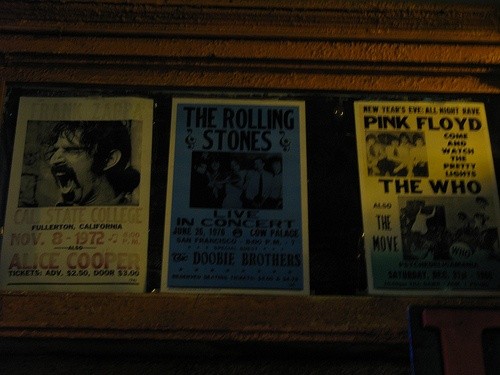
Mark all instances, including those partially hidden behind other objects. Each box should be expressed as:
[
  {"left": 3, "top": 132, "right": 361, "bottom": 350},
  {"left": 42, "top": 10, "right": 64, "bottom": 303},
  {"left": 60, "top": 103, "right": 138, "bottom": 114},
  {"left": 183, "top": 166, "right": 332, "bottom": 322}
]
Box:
[
  {"left": 41, "top": 119, "right": 141, "bottom": 208},
  {"left": 365, "top": 133, "right": 377, "bottom": 172},
  {"left": 385, "top": 137, "right": 400, "bottom": 172},
  {"left": 407, "top": 136, "right": 429, "bottom": 177},
  {"left": 395, "top": 195, "right": 500, "bottom": 297},
  {"left": 192, "top": 149, "right": 285, "bottom": 212},
  {"left": 370, "top": 134, "right": 389, "bottom": 173},
  {"left": 396, "top": 131, "right": 413, "bottom": 177}
]
